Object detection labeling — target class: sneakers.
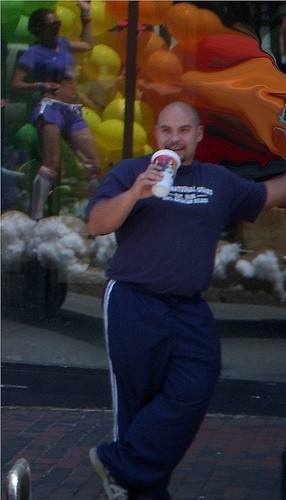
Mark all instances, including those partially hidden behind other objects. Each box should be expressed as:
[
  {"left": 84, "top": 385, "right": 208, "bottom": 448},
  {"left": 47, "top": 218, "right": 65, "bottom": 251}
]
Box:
[{"left": 90, "top": 446, "right": 128, "bottom": 500}]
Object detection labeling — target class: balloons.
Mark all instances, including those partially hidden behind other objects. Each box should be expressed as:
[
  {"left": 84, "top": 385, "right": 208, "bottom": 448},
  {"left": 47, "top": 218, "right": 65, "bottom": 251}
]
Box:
[{"left": 0, "top": 0, "right": 260, "bottom": 216}]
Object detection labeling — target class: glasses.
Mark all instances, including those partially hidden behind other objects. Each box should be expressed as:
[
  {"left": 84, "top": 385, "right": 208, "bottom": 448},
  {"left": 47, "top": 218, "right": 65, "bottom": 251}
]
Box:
[{"left": 47, "top": 20, "right": 60, "bottom": 27}]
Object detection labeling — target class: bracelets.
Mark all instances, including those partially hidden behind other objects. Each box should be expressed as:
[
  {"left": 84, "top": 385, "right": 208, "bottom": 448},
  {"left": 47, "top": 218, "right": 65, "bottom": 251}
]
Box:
[
  {"left": 81, "top": 9, "right": 91, "bottom": 16},
  {"left": 36, "top": 82, "right": 44, "bottom": 89}
]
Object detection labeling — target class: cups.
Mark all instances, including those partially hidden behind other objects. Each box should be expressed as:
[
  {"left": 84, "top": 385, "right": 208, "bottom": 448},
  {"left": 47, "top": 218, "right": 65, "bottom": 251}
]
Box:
[{"left": 145, "top": 149, "right": 181, "bottom": 198}]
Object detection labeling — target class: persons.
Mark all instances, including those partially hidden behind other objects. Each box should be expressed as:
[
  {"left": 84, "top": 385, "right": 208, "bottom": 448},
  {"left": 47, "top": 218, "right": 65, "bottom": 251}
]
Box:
[
  {"left": 11, "top": 0, "right": 101, "bottom": 221},
  {"left": 83, "top": 101, "right": 286, "bottom": 500}
]
